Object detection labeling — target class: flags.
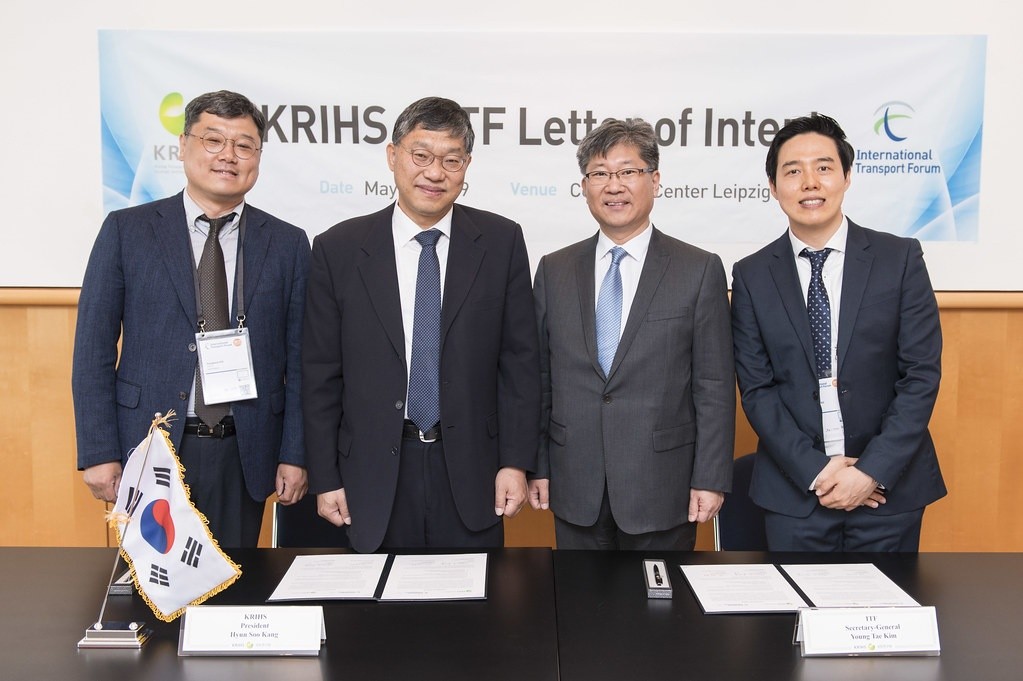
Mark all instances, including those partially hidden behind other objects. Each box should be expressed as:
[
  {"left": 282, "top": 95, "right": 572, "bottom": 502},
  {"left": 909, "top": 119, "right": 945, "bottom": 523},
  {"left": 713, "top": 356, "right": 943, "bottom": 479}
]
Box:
[{"left": 104, "top": 428, "right": 244, "bottom": 623}]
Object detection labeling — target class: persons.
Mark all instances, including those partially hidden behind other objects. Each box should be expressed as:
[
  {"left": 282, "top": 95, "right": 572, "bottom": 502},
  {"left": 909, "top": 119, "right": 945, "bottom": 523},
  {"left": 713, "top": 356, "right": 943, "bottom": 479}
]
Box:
[
  {"left": 729, "top": 115, "right": 948, "bottom": 554},
  {"left": 71, "top": 90, "right": 313, "bottom": 548},
  {"left": 533, "top": 119, "right": 735, "bottom": 549},
  {"left": 300, "top": 95, "right": 539, "bottom": 549}
]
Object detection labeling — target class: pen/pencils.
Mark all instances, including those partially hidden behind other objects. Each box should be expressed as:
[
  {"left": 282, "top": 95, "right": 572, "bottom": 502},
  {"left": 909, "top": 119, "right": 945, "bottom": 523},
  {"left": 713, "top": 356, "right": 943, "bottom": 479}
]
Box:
[{"left": 654, "top": 564, "right": 663, "bottom": 586}]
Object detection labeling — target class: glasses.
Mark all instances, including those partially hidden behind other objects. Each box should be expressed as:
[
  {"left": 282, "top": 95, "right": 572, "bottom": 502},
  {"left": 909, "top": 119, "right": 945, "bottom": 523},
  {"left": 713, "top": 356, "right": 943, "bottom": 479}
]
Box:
[
  {"left": 185, "top": 130, "right": 262, "bottom": 160},
  {"left": 397, "top": 142, "right": 469, "bottom": 173},
  {"left": 586, "top": 167, "right": 656, "bottom": 187}
]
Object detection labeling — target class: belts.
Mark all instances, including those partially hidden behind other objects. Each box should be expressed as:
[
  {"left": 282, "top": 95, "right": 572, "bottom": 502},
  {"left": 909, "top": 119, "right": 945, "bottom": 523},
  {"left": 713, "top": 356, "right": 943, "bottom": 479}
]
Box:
[
  {"left": 403, "top": 424, "right": 442, "bottom": 443},
  {"left": 184, "top": 422, "right": 236, "bottom": 439}
]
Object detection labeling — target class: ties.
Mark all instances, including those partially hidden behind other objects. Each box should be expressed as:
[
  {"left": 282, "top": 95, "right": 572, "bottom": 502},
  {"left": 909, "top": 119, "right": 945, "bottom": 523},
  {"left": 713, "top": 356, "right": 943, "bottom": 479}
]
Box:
[
  {"left": 798, "top": 248, "right": 835, "bottom": 378},
  {"left": 197, "top": 212, "right": 235, "bottom": 429},
  {"left": 596, "top": 246, "right": 628, "bottom": 380},
  {"left": 407, "top": 228, "right": 443, "bottom": 433}
]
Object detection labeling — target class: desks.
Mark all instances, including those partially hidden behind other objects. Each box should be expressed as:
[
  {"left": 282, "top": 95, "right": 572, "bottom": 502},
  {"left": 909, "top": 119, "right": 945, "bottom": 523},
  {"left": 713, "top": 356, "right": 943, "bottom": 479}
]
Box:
[{"left": 0, "top": 546, "right": 1023, "bottom": 681}]
